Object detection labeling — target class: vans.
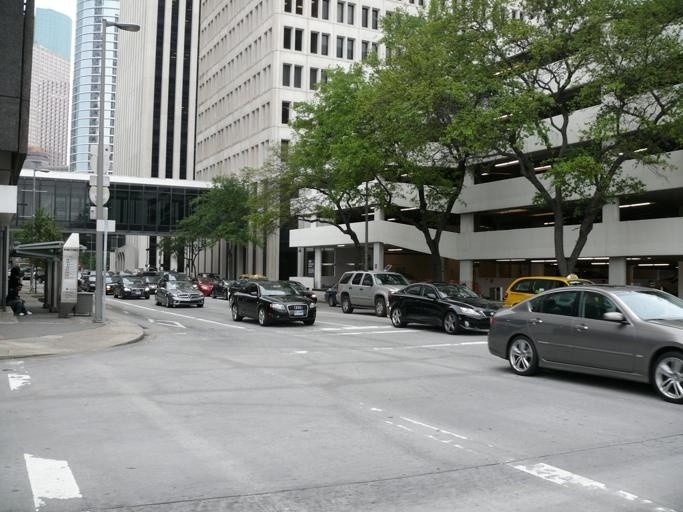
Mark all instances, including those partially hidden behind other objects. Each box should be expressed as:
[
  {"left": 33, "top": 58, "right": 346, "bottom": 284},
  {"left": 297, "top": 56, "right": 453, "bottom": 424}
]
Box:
[{"left": 503, "top": 273, "right": 598, "bottom": 312}]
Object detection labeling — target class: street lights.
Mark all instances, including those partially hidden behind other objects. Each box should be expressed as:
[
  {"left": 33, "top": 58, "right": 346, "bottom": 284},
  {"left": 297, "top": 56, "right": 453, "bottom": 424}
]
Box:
[{"left": 94, "top": 19, "right": 139, "bottom": 323}]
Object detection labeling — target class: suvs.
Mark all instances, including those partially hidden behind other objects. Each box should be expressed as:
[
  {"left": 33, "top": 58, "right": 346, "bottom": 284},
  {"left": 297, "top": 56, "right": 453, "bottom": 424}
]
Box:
[{"left": 336, "top": 272, "right": 407, "bottom": 316}]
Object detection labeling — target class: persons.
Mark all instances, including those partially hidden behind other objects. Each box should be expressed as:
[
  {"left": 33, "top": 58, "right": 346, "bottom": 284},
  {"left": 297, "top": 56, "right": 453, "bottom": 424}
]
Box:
[{"left": 5, "top": 282, "right": 32, "bottom": 316}]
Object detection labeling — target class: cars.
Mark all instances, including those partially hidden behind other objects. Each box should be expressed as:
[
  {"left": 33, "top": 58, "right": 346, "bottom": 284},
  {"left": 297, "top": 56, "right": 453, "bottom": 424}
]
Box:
[
  {"left": 488, "top": 285, "right": 682, "bottom": 404},
  {"left": 324, "top": 281, "right": 338, "bottom": 308},
  {"left": 228, "top": 282, "right": 315, "bottom": 325},
  {"left": 80, "top": 271, "right": 265, "bottom": 307},
  {"left": 389, "top": 281, "right": 500, "bottom": 333},
  {"left": 285, "top": 281, "right": 316, "bottom": 302}
]
